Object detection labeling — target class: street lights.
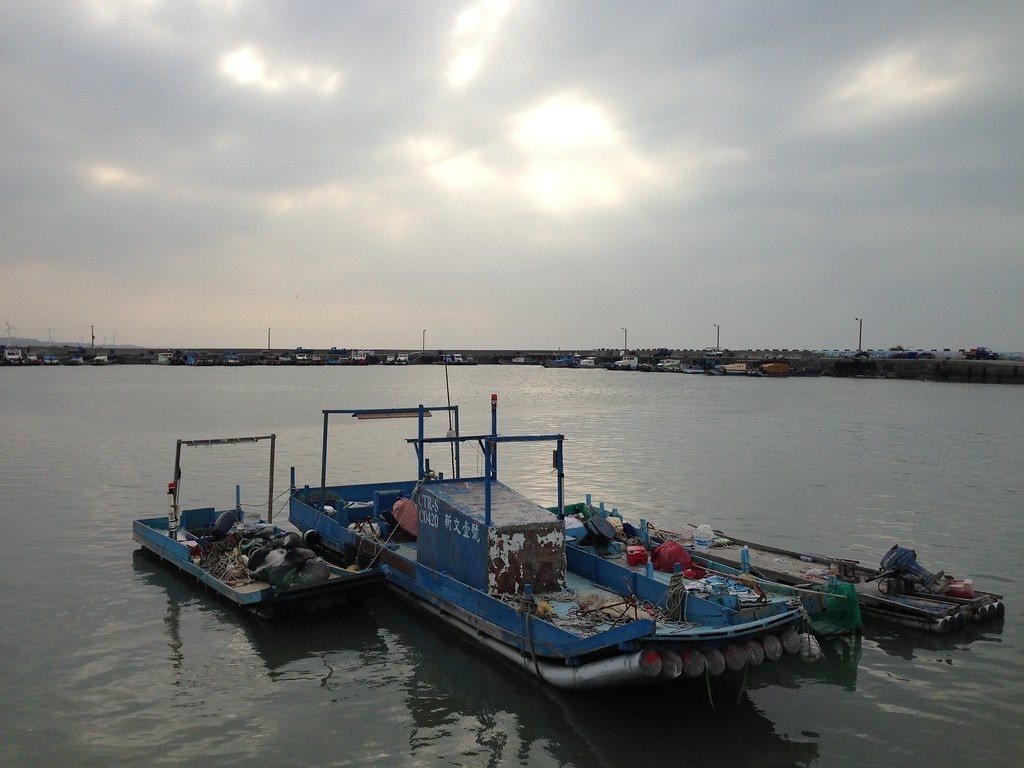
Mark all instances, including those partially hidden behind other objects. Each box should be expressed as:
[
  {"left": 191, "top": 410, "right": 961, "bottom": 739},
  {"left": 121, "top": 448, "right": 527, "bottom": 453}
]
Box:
[
  {"left": 268, "top": 327, "right": 272, "bottom": 350},
  {"left": 713, "top": 324, "right": 719, "bottom": 348},
  {"left": 854, "top": 317, "right": 862, "bottom": 351},
  {"left": 423, "top": 329, "right": 427, "bottom": 351},
  {"left": 621, "top": 328, "right": 627, "bottom": 350}
]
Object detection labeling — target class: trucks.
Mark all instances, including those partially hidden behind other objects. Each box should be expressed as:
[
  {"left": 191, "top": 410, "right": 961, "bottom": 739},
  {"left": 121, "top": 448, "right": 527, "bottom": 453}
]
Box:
[
  {"left": 962, "top": 346, "right": 1000, "bottom": 360},
  {"left": 705, "top": 348, "right": 723, "bottom": 357}
]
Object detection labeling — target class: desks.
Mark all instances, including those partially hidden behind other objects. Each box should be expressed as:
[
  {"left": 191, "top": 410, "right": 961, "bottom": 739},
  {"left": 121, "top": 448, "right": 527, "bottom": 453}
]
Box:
[{"left": 347, "top": 502, "right": 376, "bottom": 535}]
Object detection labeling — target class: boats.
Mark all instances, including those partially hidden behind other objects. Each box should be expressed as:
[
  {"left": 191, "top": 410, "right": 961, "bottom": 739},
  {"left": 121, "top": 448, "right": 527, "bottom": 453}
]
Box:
[
  {"left": 498, "top": 355, "right": 796, "bottom": 378},
  {"left": 151, "top": 351, "right": 479, "bottom": 365},
  {"left": 132, "top": 433, "right": 392, "bottom": 624},
  {"left": 286, "top": 358, "right": 826, "bottom": 691},
  {"left": 4, "top": 349, "right": 110, "bottom": 366},
  {"left": 652, "top": 523, "right": 1005, "bottom": 637}
]
